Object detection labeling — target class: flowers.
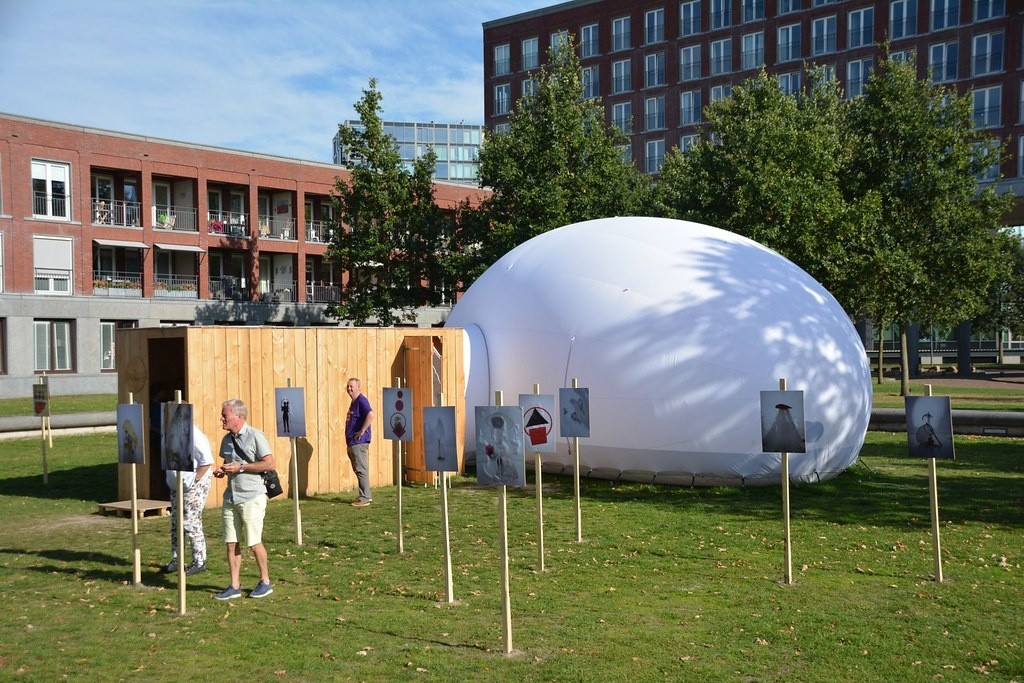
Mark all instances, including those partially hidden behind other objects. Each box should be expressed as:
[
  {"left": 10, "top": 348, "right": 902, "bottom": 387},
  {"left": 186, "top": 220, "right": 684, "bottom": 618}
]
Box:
[{"left": 93, "top": 279, "right": 196, "bottom": 293}]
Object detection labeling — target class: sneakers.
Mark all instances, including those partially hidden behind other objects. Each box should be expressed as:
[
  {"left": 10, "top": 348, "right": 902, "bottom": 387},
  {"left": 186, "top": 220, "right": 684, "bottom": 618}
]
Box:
[
  {"left": 248, "top": 580, "right": 273, "bottom": 598},
  {"left": 185, "top": 559, "right": 206, "bottom": 576},
  {"left": 214, "top": 583, "right": 242, "bottom": 601},
  {"left": 162, "top": 557, "right": 179, "bottom": 573}
]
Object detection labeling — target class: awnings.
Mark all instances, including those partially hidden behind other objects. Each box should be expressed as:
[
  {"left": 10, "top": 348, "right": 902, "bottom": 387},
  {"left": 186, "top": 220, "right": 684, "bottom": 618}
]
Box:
[
  {"left": 154, "top": 243, "right": 206, "bottom": 252},
  {"left": 93, "top": 239, "right": 150, "bottom": 249}
]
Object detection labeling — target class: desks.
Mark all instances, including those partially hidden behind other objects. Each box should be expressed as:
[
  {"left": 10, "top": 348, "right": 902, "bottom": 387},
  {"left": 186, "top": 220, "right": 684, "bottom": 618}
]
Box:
[
  {"left": 223, "top": 223, "right": 243, "bottom": 235},
  {"left": 94, "top": 209, "right": 113, "bottom": 224}
]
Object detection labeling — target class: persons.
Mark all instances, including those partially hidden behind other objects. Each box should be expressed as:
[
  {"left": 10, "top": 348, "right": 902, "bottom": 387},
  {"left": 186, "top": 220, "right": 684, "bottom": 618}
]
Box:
[
  {"left": 345, "top": 378, "right": 375, "bottom": 508},
  {"left": 158, "top": 400, "right": 214, "bottom": 576},
  {"left": 213, "top": 399, "right": 275, "bottom": 600}
]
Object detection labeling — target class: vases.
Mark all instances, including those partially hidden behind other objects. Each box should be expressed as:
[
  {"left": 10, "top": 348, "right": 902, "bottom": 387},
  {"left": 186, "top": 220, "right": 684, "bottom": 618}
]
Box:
[{"left": 94, "top": 287, "right": 197, "bottom": 298}]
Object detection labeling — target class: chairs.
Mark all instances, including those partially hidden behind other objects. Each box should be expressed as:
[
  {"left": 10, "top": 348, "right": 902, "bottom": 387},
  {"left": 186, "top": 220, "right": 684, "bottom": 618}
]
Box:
[{"left": 153, "top": 214, "right": 177, "bottom": 229}]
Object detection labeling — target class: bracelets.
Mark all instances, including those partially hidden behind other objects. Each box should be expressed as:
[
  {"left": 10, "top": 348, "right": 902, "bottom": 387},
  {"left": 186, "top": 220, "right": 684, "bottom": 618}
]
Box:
[{"left": 195, "top": 479, "right": 199, "bottom": 483}]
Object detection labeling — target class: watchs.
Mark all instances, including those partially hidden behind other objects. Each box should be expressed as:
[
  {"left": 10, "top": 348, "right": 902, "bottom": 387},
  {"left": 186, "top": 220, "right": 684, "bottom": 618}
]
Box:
[{"left": 239, "top": 463, "right": 244, "bottom": 473}]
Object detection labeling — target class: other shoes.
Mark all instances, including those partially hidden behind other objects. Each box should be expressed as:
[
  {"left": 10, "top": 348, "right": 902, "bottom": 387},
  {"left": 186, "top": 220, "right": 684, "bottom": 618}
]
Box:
[{"left": 350, "top": 496, "right": 373, "bottom": 506}]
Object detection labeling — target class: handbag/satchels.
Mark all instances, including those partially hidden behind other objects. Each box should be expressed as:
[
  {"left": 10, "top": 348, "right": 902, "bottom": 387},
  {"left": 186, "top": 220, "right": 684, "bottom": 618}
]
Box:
[{"left": 265, "top": 469, "right": 283, "bottom": 499}]
